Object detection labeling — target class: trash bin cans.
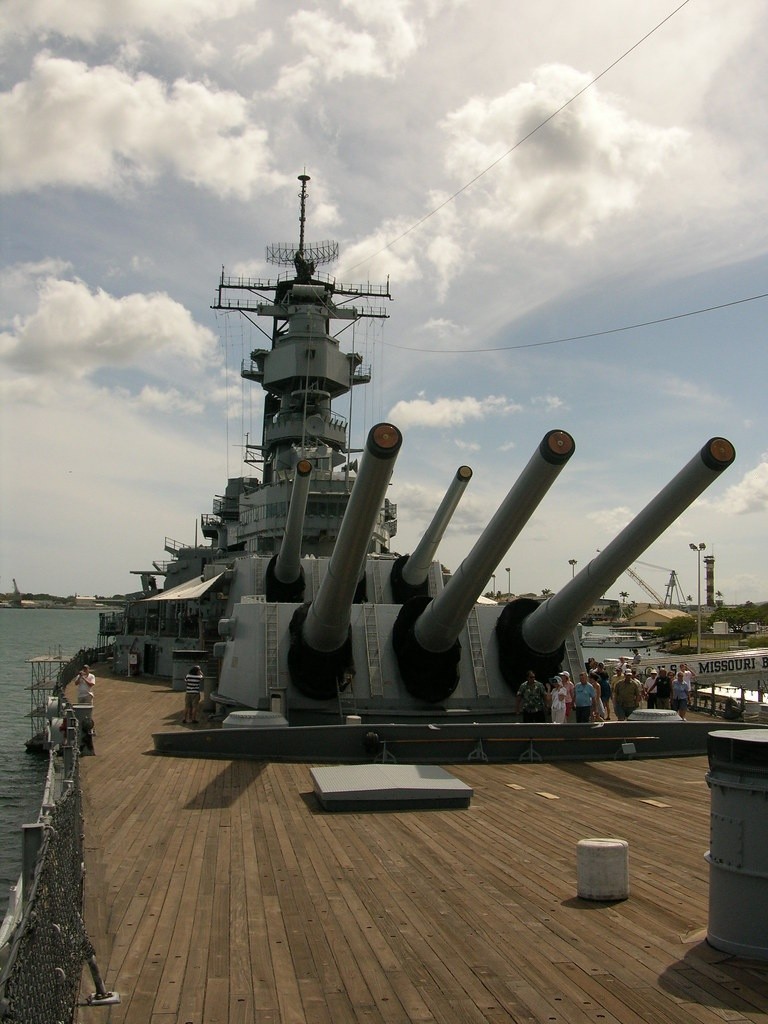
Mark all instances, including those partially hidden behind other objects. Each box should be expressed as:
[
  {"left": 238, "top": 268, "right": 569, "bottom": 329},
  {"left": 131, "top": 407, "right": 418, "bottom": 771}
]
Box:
[{"left": 697, "top": 729, "right": 768, "bottom": 959}]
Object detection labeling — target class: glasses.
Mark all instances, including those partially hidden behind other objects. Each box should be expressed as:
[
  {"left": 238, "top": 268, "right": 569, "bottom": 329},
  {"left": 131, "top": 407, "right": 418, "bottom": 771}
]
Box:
[
  {"left": 530, "top": 676, "right": 535, "bottom": 679},
  {"left": 578, "top": 686, "right": 586, "bottom": 692}
]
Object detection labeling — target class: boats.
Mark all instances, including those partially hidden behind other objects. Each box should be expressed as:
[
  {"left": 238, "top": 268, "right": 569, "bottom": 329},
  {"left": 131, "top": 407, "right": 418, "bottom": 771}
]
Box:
[{"left": 580, "top": 631, "right": 659, "bottom": 649}]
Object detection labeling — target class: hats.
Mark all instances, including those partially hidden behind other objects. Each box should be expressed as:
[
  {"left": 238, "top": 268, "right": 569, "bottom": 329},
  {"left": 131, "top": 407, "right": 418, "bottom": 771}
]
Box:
[
  {"left": 549, "top": 676, "right": 563, "bottom": 686},
  {"left": 651, "top": 670, "right": 657, "bottom": 673},
  {"left": 625, "top": 669, "right": 632, "bottom": 675},
  {"left": 558, "top": 671, "right": 570, "bottom": 677}
]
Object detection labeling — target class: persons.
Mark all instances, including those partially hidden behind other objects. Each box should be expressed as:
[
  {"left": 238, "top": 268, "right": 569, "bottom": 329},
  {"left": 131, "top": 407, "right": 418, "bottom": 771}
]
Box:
[
  {"left": 514, "top": 647, "right": 696, "bottom": 723},
  {"left": 182, "top": 665, "right": 203, "bottom": 724},
  {"left": 75, "top": 664, "right": 95, "bottom": 718}
]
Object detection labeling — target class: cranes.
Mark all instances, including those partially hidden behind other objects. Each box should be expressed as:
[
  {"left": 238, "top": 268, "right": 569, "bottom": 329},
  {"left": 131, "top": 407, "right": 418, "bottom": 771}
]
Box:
[{"left": 595, "top": 549, "right": 671, "bottom": 610}]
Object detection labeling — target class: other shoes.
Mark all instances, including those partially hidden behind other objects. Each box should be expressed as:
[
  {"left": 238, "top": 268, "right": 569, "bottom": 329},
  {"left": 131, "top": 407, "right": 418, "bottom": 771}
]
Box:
[
  {"left": 183, "top": 720, "right": 186, "bottom": 723},
  {"left": 192, "top": 720, "right": 199, "bottom": 724}
]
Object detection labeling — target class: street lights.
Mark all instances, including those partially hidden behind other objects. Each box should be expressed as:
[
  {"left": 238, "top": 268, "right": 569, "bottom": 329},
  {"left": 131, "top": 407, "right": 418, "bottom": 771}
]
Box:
[
  {"left": 568, "top": 558, "right": 578, "bottom": 577},
  {"left": 505, "top": 567, "right": 511, "bottom": 594},
  {"left": 491, "top": 574, "right": 496, "bottom": 595},
  {"left": 688, "top": 543, "right": 707, "bottom": 654}
]
